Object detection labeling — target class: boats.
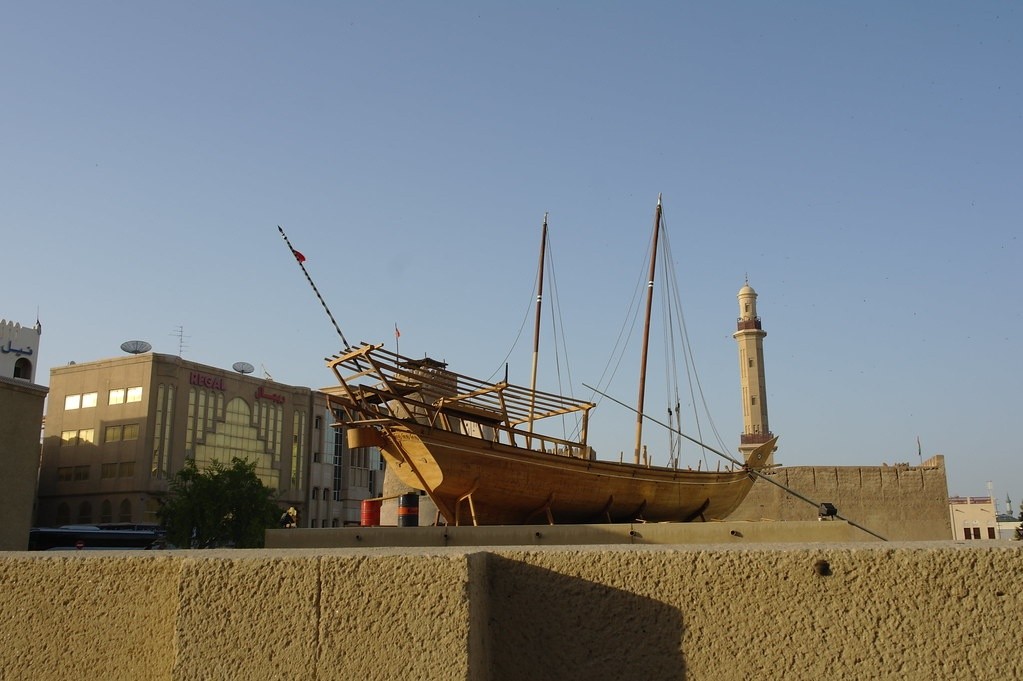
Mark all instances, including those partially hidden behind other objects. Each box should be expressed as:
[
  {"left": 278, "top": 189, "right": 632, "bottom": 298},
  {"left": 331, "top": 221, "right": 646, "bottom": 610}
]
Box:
[{"left": 275, "top": 194, "right": 780, "bottom": 525}]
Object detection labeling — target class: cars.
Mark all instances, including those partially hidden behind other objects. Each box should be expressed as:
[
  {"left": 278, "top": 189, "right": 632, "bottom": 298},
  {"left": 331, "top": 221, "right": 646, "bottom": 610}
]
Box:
[{"left": 42, "top": 546, "right": 163, "bottom": 551}]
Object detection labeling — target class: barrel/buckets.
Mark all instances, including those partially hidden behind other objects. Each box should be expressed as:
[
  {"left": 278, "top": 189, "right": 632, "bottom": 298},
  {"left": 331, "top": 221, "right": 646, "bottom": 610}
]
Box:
[
  {"left": 398, "top": 494, "right": 419, "bottom": 526},
  {"left": 361, "top": 500, "right": 380, "bottom": 527}
]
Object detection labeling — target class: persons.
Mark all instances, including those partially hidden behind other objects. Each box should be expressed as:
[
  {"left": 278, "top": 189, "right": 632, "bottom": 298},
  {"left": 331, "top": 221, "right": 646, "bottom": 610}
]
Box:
[{"left": 280, "top": 506, "right": 297, "bottom": 528}]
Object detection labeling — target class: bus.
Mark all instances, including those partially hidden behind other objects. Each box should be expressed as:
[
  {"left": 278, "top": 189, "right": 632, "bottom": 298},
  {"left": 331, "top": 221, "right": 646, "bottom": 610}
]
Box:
[{"left": 28, "top": 524, "right": 166, "bottom": 552}]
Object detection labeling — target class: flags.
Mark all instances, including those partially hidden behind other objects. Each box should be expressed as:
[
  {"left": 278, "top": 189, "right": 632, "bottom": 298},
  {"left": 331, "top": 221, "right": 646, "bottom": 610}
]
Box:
[
  {"left": 294, "top": 250, "right": 305, "bottom": 262},
  {"left": 396, "top": 328, "right": 400, "bottom": 337}
]
